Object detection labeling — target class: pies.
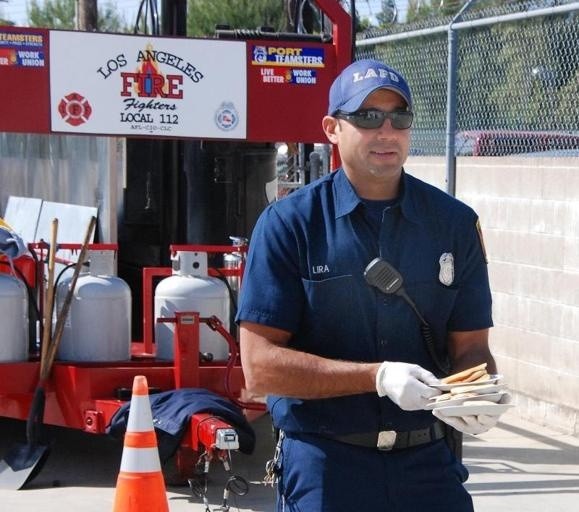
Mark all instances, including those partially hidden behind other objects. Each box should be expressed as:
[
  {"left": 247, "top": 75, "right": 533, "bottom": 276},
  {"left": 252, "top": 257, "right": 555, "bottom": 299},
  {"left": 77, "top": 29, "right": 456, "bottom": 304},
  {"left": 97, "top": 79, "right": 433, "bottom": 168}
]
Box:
[{"left": 436, "top": 363, "right": 496, "bottom": 410}]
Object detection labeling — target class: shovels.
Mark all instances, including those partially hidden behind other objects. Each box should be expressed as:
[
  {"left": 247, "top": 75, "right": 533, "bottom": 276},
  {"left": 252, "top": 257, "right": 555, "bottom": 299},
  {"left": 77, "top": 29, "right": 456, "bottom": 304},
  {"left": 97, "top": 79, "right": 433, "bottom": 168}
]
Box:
[{"left": 0, "top": 216, "right": 97, "bottom": 490}]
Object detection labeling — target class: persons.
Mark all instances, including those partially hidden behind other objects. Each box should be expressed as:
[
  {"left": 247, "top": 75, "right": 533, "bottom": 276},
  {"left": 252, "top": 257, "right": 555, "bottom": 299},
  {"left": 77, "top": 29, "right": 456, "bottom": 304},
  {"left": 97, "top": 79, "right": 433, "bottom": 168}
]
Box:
[{"left": 234, "top": 58, "right": 511, "bottom": 512}]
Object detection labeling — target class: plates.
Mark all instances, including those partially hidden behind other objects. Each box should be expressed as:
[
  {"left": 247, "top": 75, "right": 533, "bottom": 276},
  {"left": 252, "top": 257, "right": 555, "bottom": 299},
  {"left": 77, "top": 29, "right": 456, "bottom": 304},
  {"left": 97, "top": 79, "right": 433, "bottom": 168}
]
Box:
[{"left": 427, "top": 372, "right": 513, "bottom": 418}]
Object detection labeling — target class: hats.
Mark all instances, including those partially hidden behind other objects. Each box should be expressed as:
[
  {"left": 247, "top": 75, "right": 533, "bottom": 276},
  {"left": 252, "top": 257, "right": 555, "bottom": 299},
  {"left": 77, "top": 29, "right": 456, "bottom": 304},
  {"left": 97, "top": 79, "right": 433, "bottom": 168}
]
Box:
[{"left": 328, "top": 60, "right": 412, "bottom": 117}]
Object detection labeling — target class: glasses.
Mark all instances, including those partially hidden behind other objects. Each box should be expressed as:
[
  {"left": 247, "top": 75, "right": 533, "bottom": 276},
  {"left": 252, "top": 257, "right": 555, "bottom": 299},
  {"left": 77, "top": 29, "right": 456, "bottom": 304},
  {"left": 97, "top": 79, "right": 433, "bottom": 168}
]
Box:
[{"left": 335, "top": 112, "right": 413, "bottom": 129}]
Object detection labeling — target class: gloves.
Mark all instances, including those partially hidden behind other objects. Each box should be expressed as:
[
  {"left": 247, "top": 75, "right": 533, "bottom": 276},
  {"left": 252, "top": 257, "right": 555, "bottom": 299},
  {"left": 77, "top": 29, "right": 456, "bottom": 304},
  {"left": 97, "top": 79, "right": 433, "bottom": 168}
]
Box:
[{"left": 376, "top": 360, "right": 510, "bottom": 435}]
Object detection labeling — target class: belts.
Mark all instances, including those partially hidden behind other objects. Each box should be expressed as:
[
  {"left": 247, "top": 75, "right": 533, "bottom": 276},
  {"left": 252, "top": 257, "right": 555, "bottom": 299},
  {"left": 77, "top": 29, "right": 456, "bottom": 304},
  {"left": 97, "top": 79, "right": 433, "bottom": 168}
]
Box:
[{"left": 333, "top": 422, "right": 445, "bottom": 452}]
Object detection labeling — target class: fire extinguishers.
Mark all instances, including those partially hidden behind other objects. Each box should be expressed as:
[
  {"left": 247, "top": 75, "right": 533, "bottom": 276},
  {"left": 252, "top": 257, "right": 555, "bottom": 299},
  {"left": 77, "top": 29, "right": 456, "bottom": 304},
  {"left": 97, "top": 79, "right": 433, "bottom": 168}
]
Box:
[{"left": 223, "top": 236, "right": 248, "bottom": 291}]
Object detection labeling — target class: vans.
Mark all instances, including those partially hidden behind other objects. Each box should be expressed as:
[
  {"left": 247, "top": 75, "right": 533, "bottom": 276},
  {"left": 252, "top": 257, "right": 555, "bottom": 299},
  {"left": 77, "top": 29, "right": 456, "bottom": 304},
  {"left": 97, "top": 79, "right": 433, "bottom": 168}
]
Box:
[{"left": 456, "top": 132, "right": 579, "bottom": 158}]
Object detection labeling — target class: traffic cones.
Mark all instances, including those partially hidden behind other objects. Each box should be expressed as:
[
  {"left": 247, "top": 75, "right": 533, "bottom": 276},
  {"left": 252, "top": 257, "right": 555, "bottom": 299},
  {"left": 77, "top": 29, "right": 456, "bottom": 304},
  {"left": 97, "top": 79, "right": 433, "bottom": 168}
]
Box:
[{"left": 112, "top": 375, "right": 169, "bottom": 512}]
